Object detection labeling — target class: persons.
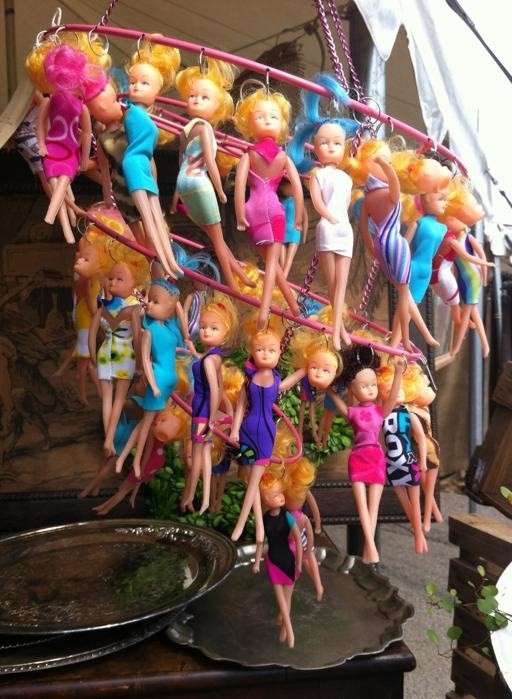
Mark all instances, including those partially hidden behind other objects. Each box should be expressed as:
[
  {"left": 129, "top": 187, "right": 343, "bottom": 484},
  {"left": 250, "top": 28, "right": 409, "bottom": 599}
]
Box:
[
  {"left": 16, "top": 30, "right": 498, "bottom": 652},
  {"left": 16, "top": 32, "right": 497, "bottom": 350},
  {"left": 53, "top": 215, "right": 443, "bottom": 647}
]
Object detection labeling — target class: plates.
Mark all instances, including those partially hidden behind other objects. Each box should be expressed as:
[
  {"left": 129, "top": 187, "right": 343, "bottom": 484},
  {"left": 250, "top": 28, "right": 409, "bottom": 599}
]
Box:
[
  {"left": 0, "top": 518, "right": 237, "bottom": 635},
  {"left": 0, "top": 605, "right": 187, "bottom": 674}
]
